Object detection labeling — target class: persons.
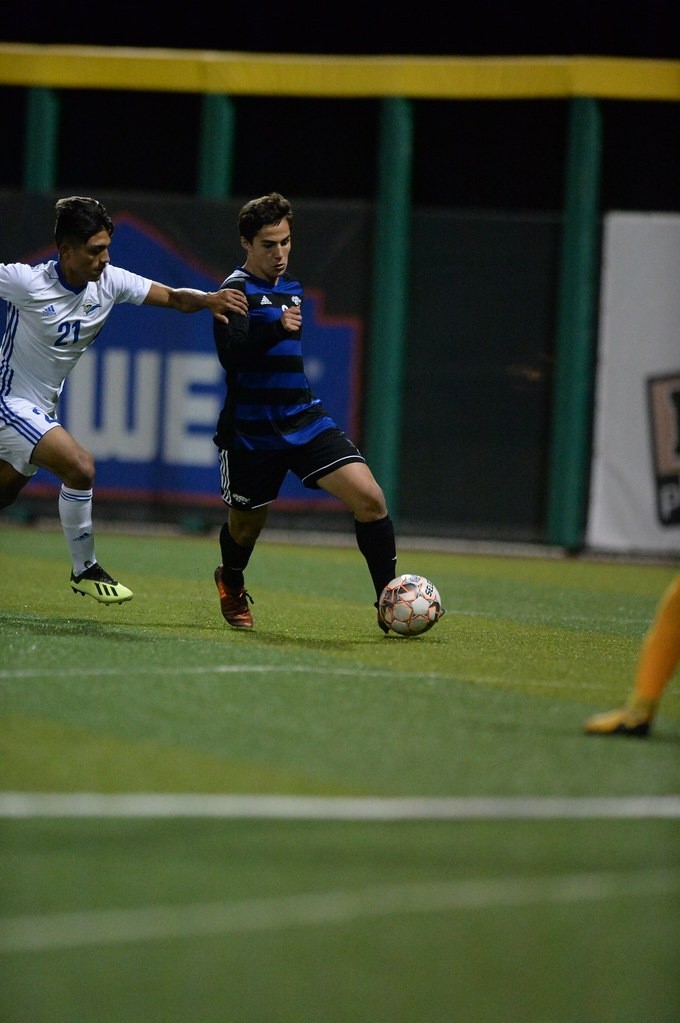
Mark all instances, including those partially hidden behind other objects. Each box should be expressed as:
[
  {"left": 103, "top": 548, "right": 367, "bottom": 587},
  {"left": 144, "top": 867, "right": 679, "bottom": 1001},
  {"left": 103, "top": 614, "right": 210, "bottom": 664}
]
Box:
[
  {"left": 0, "top": 196, "right": 248, "bottom": 605},
  {"left": 583, "top": 569, "right": 680, "bottom": 737},
  {"left": 213, "top": 192, "right": 444, "bottom": 636}
]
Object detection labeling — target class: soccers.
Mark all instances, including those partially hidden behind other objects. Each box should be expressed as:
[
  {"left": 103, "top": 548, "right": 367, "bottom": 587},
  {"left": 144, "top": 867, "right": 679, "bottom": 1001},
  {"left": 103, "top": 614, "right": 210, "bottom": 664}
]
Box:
[{"left": 378, "top": 573, "right": 442, "bottom": 634}]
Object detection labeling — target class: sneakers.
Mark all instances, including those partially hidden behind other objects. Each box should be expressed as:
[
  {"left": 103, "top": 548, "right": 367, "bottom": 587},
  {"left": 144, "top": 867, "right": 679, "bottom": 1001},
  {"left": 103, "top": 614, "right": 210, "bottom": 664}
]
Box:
[
  {"left": 69, "top": 559, "right": 134, "bottom": 606},
  {"left": 214, "top": 565, "right": 255, "bottom": 628},
  {"left": 374, "top": 598, "right": 446, "bottom": 633}
]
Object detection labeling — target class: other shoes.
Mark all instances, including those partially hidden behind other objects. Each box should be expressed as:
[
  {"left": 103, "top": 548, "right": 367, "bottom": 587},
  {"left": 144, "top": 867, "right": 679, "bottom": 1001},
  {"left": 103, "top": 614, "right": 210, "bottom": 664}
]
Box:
[{"left": 583, "top": 707, "right": 651, "bottom": 736}]
